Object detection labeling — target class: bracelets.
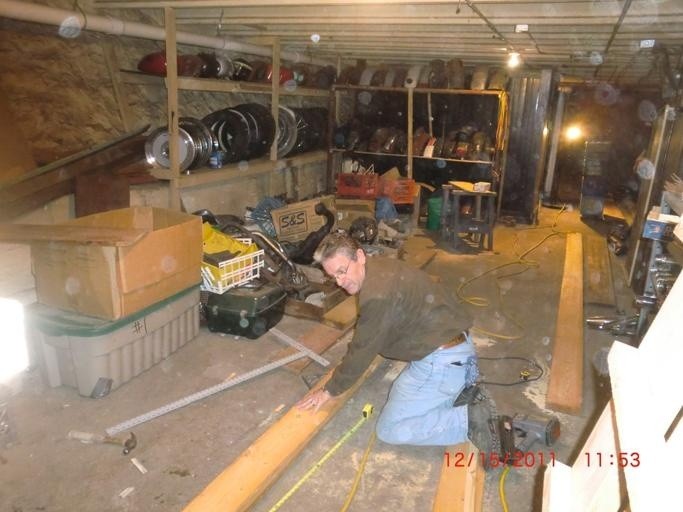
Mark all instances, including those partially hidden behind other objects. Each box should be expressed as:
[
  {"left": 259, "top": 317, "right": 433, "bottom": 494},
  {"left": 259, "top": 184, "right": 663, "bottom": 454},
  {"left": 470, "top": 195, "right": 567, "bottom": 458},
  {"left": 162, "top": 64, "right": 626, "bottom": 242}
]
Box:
[{"left": 321, "top": 385, "right": 331, "bottom": 397}]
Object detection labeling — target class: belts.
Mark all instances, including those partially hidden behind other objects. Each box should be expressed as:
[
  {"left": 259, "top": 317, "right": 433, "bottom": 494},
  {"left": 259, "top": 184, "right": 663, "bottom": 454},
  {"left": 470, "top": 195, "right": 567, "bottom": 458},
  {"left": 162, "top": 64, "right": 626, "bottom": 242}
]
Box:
[{"left": 439, "top": 328, "right": 469, "bottom": 349}]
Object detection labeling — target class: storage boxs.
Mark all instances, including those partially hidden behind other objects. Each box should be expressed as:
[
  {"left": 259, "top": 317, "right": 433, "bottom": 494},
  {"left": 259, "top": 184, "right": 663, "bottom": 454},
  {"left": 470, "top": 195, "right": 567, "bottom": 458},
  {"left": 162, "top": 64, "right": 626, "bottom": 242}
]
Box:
[
  {"left": 29, "top": 203, "right": 203, "bottom": 321},
  {"left": 270, "top": 195, "right": 337, "bottom": 243},
  {"left": 334, "top": 196, "right": 378, "bottom": 231},
  {"left": 206, "top": 282, "right": 286, "bottom": 340},
  {"left": 23, "top": 282, "right": 202, "bottom": 397},
  {"left": 378, "top": 176, "right": 414, "bottom": 205},
  {"left": 337, "top": 173, "right": 378, "bottom": 198}
]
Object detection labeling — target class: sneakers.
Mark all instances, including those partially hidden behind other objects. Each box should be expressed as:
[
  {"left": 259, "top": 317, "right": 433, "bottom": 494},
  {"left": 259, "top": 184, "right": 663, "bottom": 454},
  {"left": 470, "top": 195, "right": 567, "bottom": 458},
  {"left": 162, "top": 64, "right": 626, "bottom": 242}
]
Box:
[{"left": 455, "top": 384, "right": 499, "bottom": 470}]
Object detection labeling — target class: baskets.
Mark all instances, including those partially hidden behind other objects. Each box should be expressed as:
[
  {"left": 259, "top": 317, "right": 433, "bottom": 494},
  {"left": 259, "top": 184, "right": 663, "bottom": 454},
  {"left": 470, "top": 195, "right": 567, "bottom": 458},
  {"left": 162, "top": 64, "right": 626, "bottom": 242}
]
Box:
[{"left": 336, "top": 172, "right": 415, "bottom": 204}]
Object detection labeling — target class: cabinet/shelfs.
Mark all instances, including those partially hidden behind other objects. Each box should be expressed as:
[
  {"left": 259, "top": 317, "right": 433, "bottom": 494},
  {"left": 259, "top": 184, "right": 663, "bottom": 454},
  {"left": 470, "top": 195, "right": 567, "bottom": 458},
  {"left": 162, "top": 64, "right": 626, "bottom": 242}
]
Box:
[
  {"left": 634, "top": 191, "right": 681, "bottom": 346},
  {"left": 326, "top": 84, "right": 512, "bottom": 226}
]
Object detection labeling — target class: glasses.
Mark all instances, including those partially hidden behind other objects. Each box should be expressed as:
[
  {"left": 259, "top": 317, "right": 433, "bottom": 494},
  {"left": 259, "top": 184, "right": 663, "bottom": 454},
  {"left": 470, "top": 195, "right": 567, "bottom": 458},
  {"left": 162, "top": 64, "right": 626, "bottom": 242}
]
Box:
[{"left": 329, "top": 255, "right": 352, "bottom": 283}]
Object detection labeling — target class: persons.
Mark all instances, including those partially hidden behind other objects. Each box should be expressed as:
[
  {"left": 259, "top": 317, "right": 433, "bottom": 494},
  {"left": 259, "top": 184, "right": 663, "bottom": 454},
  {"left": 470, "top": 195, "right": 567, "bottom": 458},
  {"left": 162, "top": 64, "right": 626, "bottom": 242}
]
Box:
[{"left": 293, "top": 231, "right": 516, "bottom": 467}]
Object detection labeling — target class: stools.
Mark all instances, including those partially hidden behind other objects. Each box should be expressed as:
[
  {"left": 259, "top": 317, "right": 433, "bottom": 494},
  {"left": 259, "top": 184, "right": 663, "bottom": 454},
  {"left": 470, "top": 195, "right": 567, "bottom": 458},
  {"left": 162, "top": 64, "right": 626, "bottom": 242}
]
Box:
[
  {"left": 441, "top": 184, "right": 481, "bottom": 242},
  {"left": 452, "top": 189, "right": 497, "bottom": 252}
]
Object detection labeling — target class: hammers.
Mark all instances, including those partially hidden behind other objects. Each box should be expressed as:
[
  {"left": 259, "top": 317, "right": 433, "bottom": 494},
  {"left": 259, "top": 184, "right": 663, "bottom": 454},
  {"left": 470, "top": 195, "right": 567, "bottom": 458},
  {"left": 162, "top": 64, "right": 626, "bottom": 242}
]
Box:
[{"left": 63, "top": 432, "right": 136, "bottom": 454}]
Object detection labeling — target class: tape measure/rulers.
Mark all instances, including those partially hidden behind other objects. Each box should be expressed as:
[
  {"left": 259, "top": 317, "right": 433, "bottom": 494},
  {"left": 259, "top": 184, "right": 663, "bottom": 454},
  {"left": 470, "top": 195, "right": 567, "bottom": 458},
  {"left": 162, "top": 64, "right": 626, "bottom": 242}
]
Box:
[
  {"left": 105, "top": 327, "right": 330, "bottom": 435},
  {"left": 267, "top": 403, "right": 374, "bottom": 511}
]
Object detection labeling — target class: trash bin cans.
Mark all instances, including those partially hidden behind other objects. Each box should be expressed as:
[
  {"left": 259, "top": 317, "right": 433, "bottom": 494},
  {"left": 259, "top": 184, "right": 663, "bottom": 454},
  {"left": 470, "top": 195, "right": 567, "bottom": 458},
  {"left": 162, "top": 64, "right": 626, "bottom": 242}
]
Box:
[{"left": 427, "top": 197, "right": 451, "bottom": 230}]
